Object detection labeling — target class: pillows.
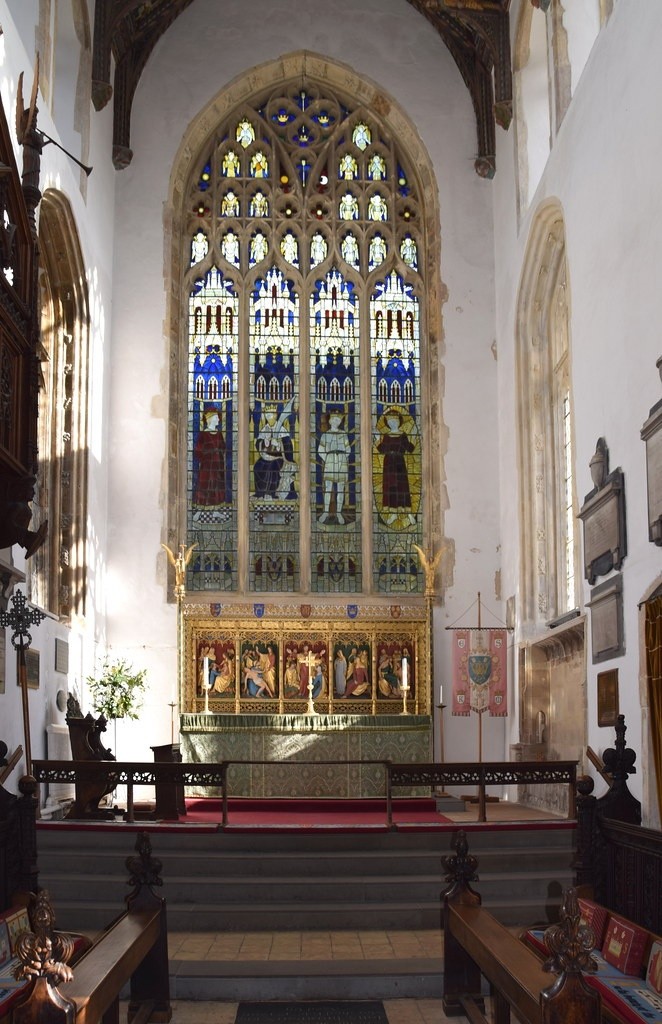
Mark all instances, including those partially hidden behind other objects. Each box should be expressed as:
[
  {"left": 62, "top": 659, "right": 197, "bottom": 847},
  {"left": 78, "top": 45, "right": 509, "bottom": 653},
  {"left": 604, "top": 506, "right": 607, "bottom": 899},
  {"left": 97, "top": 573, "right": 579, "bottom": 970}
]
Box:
[
  {"left": 601, "top": 916, "right": 647, "bottom": 975},
  {"left": 577, "top": 898, "right": 607, "bottom": 950},
  {"left": 4, "top": 903, "right": 31, "bottom": 959},
  {"left": 645, "top": 940, "right": 662, "bottom": 998},
  {"left": 0, "top": 918, "right": 11, "bottom": 968}
]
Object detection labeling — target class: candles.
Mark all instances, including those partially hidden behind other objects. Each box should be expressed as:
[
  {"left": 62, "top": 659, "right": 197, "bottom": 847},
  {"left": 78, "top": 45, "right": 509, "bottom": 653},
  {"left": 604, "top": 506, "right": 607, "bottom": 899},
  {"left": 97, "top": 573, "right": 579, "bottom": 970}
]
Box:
[
  {"left": 440, "top": 685, "right": 442, "bottom": 703},
  {"left": 401, "top": 658, "right": 408, "bottom": 687},
  {"left": 203, "top": 657, "right": 209, "bottom": 684}
]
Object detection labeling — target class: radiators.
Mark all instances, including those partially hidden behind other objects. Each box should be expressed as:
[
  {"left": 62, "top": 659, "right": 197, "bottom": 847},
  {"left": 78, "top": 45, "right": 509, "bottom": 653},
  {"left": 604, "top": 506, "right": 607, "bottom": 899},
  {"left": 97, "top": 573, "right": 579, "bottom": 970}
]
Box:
[{"left": 46, "top": 725, "right": 75, "bottom": 803}]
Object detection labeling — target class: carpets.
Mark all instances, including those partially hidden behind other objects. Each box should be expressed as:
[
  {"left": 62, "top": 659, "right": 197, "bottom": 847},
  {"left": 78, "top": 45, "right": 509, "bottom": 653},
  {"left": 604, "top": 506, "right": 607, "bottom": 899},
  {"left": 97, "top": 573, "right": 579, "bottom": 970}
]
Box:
[{"left": 235, "top": 998, "right": 388, "bottom": 1024}]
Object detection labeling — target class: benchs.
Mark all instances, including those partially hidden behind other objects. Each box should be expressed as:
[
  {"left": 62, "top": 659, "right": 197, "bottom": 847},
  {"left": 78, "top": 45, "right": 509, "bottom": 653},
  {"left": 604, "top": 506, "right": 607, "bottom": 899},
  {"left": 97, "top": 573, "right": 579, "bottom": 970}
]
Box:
[
  {"left": 0, "top": 769, "right": 89, "bottom": 1024},
  {"left": 522, "top": 775, "right": 662, "bottom": 1024},
  {"left": 65, "top": 692, "right": 125, "bottom": 820}
]
